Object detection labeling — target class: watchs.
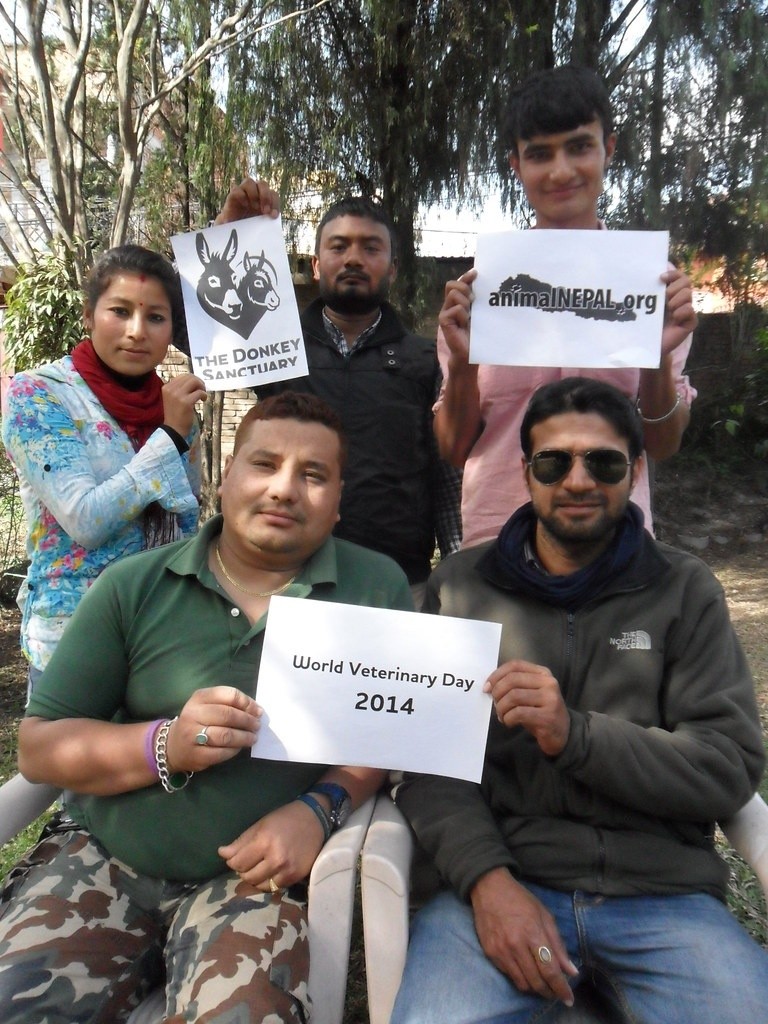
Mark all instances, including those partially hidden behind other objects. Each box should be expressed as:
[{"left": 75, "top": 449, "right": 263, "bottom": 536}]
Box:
[{"left": 309, "top": 782, "right": 352, "bottom": 828}]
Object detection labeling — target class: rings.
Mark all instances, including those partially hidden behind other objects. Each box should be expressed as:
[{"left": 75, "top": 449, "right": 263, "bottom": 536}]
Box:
[
  {"left": 532, "top": 944, "right": 555, "bottom": 964},
  {"left": 195, "top": 725, "right": 209, "bottom": 747},
  {"left": 269, "top": 877, "right": 283, "bottom": 894}
]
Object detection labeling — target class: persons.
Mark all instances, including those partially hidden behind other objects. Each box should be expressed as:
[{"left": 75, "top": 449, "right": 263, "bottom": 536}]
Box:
[
  {"left": 1, "top": 244, "right": 208, "bottom": 713},
  {"left": 1, "top": 389, "right": 417, "bottom": 1024},
  {"left": 389, "top": 375, "right": 767, "bottom": 1023},
  {"left": 432, "top": 61, "right": 698, "bottom": 559},
  {"left": 215, "top": 177, "right": 439, "bottom": 614}
]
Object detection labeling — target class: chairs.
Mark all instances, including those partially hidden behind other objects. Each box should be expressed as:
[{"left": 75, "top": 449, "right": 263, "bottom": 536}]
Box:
[{"left": 0, "top": 773, "right": 768, "bottom": 1024}]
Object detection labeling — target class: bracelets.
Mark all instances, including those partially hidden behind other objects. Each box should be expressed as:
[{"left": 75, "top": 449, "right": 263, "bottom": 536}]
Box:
[
  {"left": 143, "top": 719, "right": 166, "bottom": 780},
  {"left": 636, "top": 392, "right": 681, "bottom": 425},
  {"left": 296, "top": 794, "right": 331, "bottom": 843},
  {"left": 156, "top": 716, "right": 194, "bottom": 794}
]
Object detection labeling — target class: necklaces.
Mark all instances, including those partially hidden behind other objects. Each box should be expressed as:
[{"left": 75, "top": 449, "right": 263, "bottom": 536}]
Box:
[{"left": 212, "top": 538, "right": 297, "bottom": 598}]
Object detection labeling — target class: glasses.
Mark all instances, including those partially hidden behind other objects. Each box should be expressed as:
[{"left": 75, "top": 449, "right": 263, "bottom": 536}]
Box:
[{"left": 524, "top": 449, "right": 634, "bottom": 485}]
